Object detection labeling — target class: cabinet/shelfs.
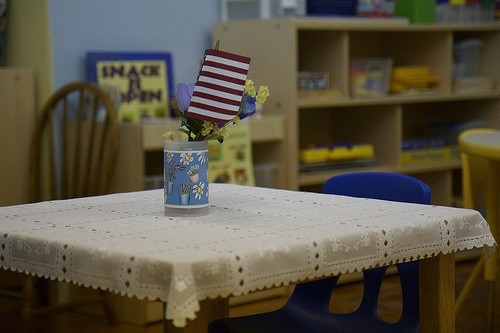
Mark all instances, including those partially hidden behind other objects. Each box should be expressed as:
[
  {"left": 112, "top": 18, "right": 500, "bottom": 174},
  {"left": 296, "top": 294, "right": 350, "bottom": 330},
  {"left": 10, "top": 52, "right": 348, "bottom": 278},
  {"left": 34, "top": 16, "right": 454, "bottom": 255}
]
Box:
[{"left": 210, "top": 18, "right": 500, "bottom": 306}]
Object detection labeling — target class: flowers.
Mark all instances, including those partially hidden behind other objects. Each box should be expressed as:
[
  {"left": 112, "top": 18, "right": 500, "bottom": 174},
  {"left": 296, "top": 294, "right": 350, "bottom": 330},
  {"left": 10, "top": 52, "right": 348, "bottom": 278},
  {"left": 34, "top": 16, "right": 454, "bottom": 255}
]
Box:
[{"left": 170, "top": 38, "right": 270, "bottom": 144}]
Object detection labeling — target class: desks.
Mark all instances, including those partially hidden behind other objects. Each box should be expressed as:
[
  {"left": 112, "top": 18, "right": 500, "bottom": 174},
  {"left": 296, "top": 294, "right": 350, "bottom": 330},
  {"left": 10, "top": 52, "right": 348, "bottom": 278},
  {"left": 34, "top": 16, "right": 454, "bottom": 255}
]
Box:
[{"left": 0, "top": 182, "right": 496, "bottom": 333}]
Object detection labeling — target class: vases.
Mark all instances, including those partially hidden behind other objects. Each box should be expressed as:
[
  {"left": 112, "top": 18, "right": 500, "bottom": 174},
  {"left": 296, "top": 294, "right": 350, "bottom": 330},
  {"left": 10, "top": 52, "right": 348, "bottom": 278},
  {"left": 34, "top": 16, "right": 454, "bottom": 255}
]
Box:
[{"left": 163, "top": 139, "right": 209, "bottom": 217}]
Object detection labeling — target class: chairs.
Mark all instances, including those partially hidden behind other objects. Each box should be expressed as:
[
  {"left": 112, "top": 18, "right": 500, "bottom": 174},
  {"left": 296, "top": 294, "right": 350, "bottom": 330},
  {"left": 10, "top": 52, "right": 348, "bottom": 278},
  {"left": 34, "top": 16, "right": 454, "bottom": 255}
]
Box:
[
  {"left": 206, "top": 173, "right": 432, "bottom": 332},
  {"left": 1, "top": 83, "right": 116, "bottom": 332}
]
